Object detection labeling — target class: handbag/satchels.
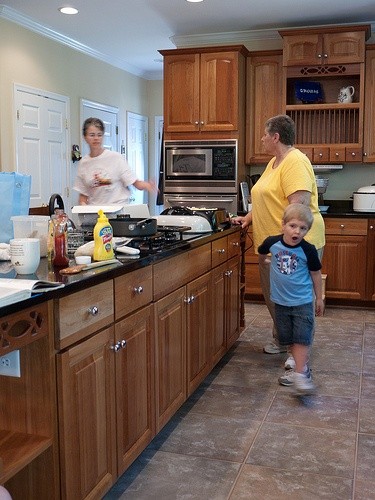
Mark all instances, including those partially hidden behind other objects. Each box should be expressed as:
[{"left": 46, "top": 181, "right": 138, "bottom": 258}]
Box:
[{"left": 0, "top": 171, "right": 32, "bottom": 243}]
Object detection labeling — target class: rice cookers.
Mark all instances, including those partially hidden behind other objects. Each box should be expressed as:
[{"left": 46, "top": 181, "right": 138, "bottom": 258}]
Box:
[{"left": 349, "top": 183, "right": 375, "bottom": 212}]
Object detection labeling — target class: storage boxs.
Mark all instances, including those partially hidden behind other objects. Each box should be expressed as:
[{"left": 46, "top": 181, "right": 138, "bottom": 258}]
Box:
[{"left": 10, "top": 215, "right": 50, "bottom": 257}]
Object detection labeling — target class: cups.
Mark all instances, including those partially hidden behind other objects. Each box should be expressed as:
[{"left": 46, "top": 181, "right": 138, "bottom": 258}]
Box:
[
  {"left": 10, "top": 239, "right": 41, "bottom": 275},
  {"left": 68, "top": 234, "right": 84, "bottom": 255}
]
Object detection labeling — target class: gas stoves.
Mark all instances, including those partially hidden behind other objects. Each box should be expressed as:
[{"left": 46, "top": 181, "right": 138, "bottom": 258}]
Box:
[{"left": 124, "top": 225, "right": 191, "bottom": 253}]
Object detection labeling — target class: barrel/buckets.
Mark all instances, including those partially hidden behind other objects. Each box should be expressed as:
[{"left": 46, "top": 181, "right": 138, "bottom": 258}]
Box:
[{"left": 10, "top": 215, "right": 50, "bottom": 257}]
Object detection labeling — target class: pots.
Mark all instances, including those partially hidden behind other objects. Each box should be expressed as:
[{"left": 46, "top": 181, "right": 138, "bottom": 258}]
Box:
[{"left": 108, "top": 214, "right": 158, "bottom": 237}]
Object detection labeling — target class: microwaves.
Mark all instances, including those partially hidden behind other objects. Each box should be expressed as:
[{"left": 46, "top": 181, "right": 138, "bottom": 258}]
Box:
[{"left": 163, "top": 139, "right": 237, "bottom": 193}]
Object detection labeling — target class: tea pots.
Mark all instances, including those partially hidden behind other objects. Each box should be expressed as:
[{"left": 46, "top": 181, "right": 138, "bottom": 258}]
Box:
[{"left": 338, "top": 85, "right": 354, "bottom": 104}]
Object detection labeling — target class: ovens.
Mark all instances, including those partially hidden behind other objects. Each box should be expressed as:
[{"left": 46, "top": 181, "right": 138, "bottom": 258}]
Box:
[{"left": 163, "top": 194, "right": 238, "bottom": 215}]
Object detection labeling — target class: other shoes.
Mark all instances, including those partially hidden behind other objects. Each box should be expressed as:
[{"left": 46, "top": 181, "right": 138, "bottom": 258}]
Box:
[
  {"left": 285, "top": 357, "right": 296, "bottom": 368},
  {"left": 278, "top": 368, "right": 312, "bottom": 385},
  {"left": 264, "top": 340, "right": 290, "bottom": 353}
]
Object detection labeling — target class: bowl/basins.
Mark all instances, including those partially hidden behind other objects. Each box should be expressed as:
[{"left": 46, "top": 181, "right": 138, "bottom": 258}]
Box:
[{"left": 319, "top": 206, "right": 329, "bottom": 211}]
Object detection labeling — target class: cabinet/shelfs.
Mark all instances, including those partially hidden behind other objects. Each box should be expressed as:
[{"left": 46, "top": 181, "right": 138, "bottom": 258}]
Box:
[
  {"left": 242, "top": 216, "right": 375, "bottom": 309},
  {"left": 0, "top": 226, "right": 246, "bottom": 500},
  {"left": 156, "top": 25, "right": 375, "bottom": 165}
]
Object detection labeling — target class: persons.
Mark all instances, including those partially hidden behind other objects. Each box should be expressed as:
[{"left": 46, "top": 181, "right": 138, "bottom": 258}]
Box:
[
  {"left": 73, "top": 118, "right": 151, "bottom": 207},
  {"left": 258, "top": 202, "right": 324, "bottom": 391},
  {"left": 230, "top": 115, "right": 326, "bottom": 368}
]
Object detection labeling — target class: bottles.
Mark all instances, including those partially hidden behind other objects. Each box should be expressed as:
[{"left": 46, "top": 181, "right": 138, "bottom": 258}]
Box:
[
  {"left": 94, "top": 210, "right": 115, "bottom": 261},
  {"left": 47, "top": 209, "right": 69, "bottom": 262},
  {"left": 52, "top": 216, "right": 70, "bottom": 267}
]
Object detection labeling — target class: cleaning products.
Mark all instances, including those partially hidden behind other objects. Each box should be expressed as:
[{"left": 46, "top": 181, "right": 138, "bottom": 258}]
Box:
[{"left": 93, "top": 209, "right": 114, "bottom": 261}]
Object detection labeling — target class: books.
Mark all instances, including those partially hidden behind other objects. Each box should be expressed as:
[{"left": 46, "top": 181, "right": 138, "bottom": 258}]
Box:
[{"left": 0, "top": 277, "right": 66, "bottom": 308}]
